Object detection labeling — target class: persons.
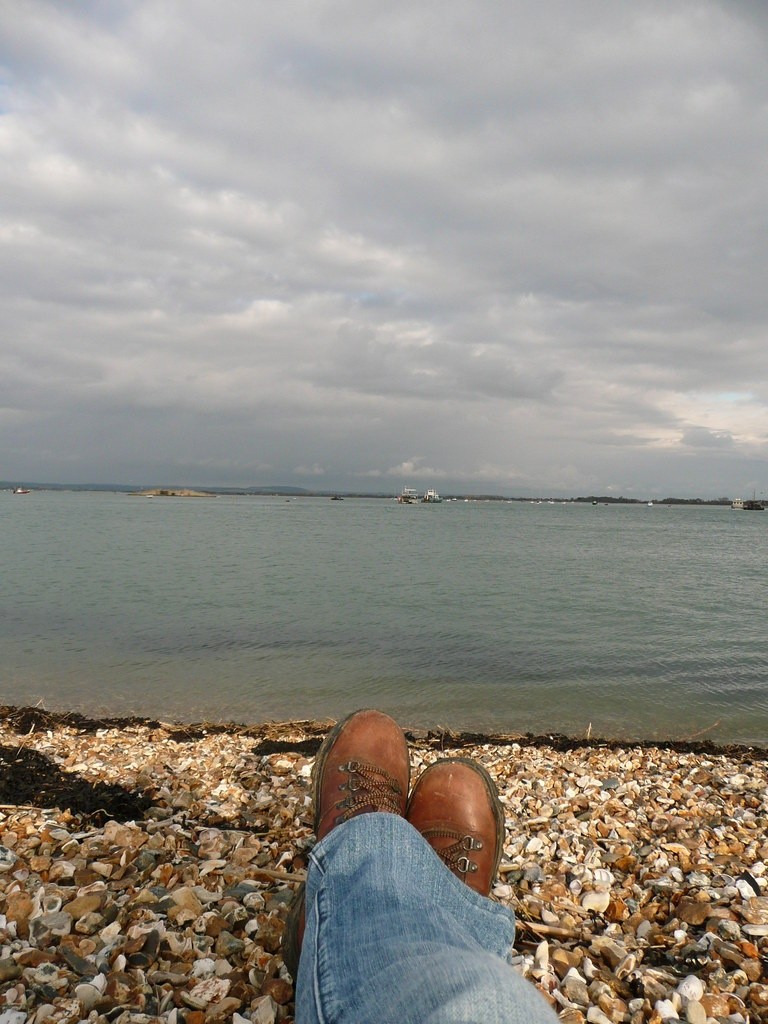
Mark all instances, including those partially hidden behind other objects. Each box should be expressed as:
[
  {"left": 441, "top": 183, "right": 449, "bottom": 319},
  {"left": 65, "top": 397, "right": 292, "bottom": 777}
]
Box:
[{"left": 283, "top": 708, "right": 565, "bottom": 1024}]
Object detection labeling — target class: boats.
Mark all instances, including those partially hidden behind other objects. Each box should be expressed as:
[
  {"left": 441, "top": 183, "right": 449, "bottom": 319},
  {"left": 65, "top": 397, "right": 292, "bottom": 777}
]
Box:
[
  {"left": 730, "top": 498, "right": 743, "bottom": 509},
  {"left": 12, "top": 487, "right": 29, "bottom": 494},
  {"left": 420, "top": 486, "right": 442, "bottom": 503},
  {"left": 742, "top": 488, "right": 765, "bottom": 510},
  {"left": 330, "top": 493, "right": 344, "bottom": 501},
  {"left": 396, "top": 483, "right": 419, "bottom": 504}
]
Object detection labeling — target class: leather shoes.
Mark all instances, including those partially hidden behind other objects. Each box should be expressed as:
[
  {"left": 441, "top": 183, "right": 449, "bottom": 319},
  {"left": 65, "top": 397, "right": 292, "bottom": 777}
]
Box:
[
  {"left": 406, "top": 757, "right": 506, "bottom": 899},
  {"left": 281, "top": 708, "right": 412, "bottom": 984}
]
